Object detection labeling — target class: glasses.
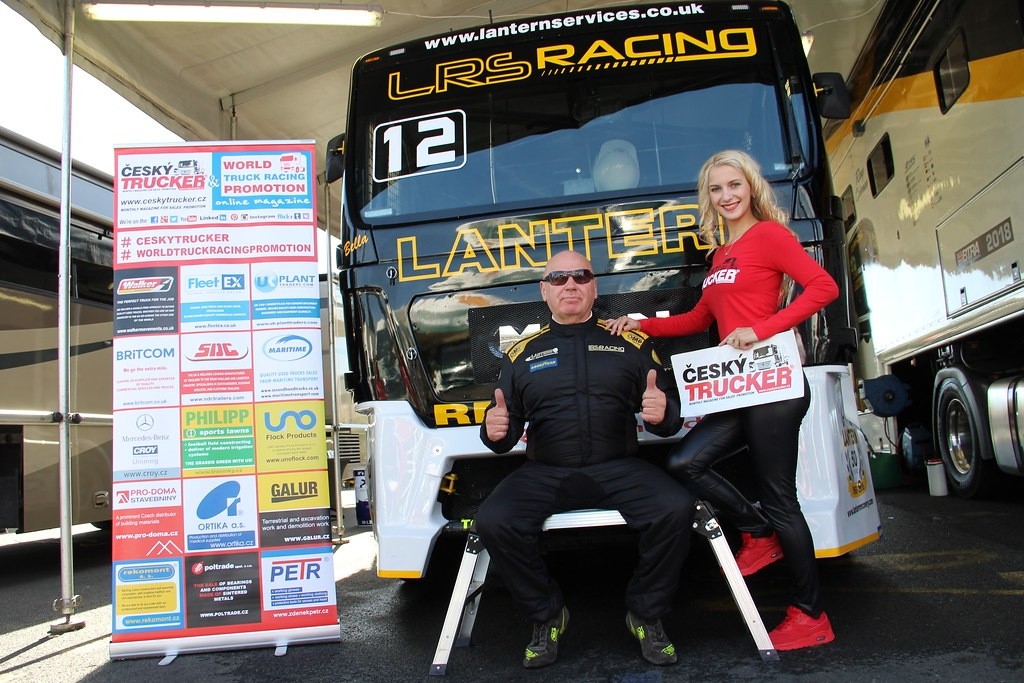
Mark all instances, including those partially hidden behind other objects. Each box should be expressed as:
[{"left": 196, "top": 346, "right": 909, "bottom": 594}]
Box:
[{"left": 542, "top": 270, "right": 594, "bottom": 286}]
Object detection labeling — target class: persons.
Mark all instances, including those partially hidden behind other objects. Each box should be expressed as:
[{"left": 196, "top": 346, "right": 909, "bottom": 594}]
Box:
[
  {"left": 602, "top": 149, "right": 838, "bottom": 654},
  {"left": 470, "top": 249, "right": 693, "bottom": 670}
]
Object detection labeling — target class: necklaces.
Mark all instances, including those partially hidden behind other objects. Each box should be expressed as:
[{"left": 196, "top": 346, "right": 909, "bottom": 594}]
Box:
[{"left": 723, "top": 216, "right": 756, "bottom": 256}]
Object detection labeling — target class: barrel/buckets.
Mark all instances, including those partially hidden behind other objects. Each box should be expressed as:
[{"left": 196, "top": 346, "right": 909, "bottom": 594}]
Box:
[{"left": 926, "top": 458, "right": 948, "bottom": 497}]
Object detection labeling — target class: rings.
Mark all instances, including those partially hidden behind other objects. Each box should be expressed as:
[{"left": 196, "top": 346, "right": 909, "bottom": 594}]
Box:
[{"left": 728, "top": 337, "right": 736, "bottom": 342}]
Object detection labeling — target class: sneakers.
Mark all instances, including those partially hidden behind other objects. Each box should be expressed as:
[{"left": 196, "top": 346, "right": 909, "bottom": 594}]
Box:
[
  {"left": 767, "top": 608, "right": 833, "bottom": 651},
  {"left": 624, "top": 609, "right": 680, "bottom": 664},
  {"left": 524, "top": 606, "right": 569, "bottom": 668},
  {"left": 719, "top": 528, "right": 781, "bottom": 576}
]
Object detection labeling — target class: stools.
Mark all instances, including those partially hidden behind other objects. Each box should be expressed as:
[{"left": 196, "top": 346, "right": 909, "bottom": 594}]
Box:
[{"left": 425, "top": 504, "right": 789, "bottom": 680}]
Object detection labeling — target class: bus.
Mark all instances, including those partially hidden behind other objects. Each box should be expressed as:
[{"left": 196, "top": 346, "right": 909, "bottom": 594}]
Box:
[
  {"left": 811, "top": 2, "right": 1022, "bottom": 534},
  {"left": 311, "top": 1, "right": 894, "bottom": 615},
  {"left": 0, "top": 128, "right": 368, "bottom": 540}
]
